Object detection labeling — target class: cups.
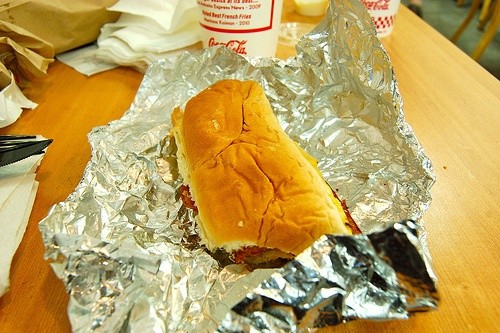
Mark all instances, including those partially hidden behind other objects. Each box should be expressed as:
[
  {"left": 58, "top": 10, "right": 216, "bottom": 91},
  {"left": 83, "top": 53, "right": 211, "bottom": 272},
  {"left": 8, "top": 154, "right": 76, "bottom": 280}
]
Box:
[
  {"left": 360, "top": 0, "right": 401, "bottom": 37},
  {"left": 199, "top": 0, "right": 282, "bottom": 64},
  {"left": 295, "top": 0, "right": 329, "bottom": 17},
  {"left": 277, "top": 22, "right": 315, "bottom": 61}
]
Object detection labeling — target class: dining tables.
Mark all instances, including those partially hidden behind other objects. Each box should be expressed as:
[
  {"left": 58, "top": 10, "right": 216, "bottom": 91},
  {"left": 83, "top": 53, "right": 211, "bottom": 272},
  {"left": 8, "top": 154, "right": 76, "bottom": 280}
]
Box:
[{"left": 0, "top": 0, "right": 500, "bottom": 333}]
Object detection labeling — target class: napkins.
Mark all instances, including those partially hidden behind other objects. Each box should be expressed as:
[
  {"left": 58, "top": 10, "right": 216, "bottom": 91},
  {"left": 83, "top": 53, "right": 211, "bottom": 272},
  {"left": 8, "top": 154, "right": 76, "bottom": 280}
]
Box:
[
  {"left": 54, "top": 0, "right": 201, "bottom": 77},
  {"left": 0, "top": 135, "right": 50, "bottom": 297}
]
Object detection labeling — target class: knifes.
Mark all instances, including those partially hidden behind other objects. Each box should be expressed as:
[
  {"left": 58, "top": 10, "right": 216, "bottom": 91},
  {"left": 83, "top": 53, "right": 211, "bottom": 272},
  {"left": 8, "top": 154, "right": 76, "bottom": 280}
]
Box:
[{"left": 0, "top": 135, "right": 54, "bottom": 168}]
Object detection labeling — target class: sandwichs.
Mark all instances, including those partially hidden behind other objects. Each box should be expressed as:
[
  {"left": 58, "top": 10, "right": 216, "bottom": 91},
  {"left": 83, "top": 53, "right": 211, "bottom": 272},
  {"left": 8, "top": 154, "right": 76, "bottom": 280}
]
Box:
[{"left": 172, "top": 79, "right": 361, "bottom": 269}]
